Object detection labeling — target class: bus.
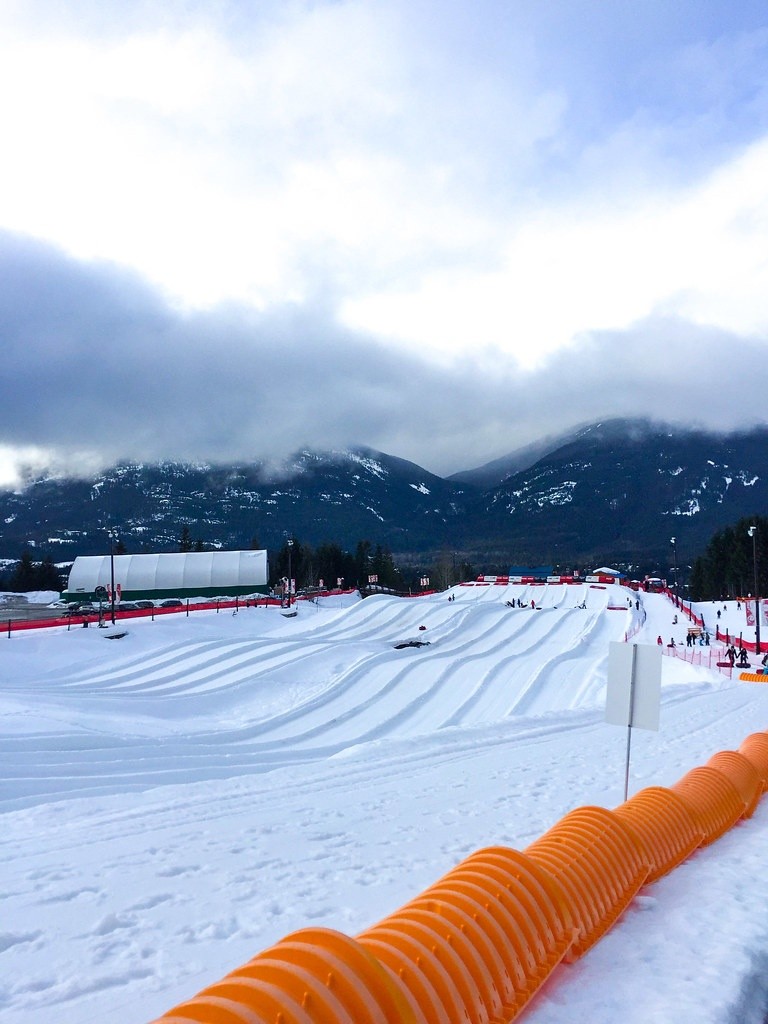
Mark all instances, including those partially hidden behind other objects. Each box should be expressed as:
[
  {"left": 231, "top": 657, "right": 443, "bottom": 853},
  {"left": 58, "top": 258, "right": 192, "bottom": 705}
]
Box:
[{"left": 643, "top": 578, "right": 663, "bottom": 593}]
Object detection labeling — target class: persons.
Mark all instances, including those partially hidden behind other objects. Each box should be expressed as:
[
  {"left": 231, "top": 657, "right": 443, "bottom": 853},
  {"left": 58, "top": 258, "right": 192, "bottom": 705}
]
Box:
[
  {"left": 629, "top": 599, "right": 639, "bottom": 611},
  {"left": 657, "top": 632, "right": 768, "bottom": 676},
  {"left": 448, "top": 594, "right": 456, "bottom": 603},
  {"left": 673, "top": 615, "right": 678, "bottom": 623},
  {"left": 580, "top": 600, "right": 587, "bottom": 609},
  {"left": 506, "top": 597, "right": 535, "bottom": 609},
  {"left": 712, "top": 592, "right": 751, "bottom": 619}
]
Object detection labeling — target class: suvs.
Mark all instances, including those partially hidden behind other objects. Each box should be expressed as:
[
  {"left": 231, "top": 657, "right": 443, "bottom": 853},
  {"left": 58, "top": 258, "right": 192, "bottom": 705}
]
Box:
[
  {"left": 163, "top": 600, "right": 181, "bottom": 607},
  {"left": 123, "top": 603, "right": 139, "bottom": 609},
  {"left": 137, "top": 601, "right": 154, "bottom": 608},
  {"left": 69, "top": 601, "right": 93, "bottom": 610},
  {"left": 79, "top": 606, "right": 99, "bottom": 614},
  {"left": 102, "top": 604, "right": 125, "bottom": 615}
]
